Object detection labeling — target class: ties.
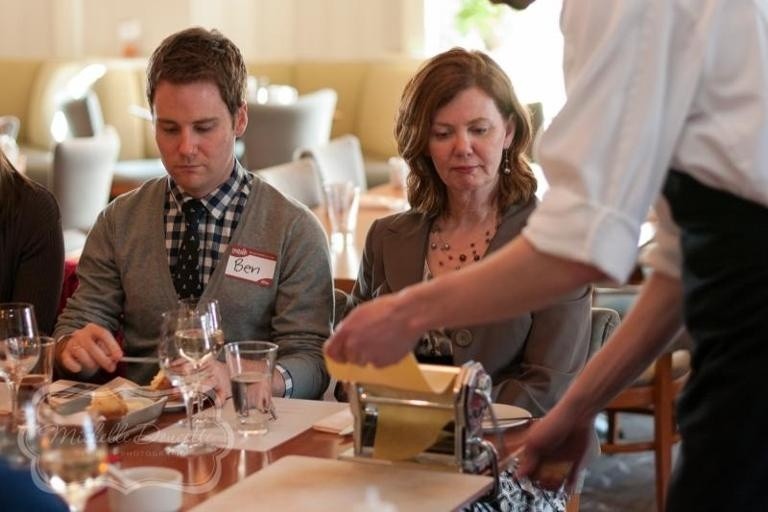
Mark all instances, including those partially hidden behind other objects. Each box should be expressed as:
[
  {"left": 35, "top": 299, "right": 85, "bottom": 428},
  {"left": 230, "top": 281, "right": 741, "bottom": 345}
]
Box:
[{"left": 174, "top": 201, "right": 204, "bottom": 299}]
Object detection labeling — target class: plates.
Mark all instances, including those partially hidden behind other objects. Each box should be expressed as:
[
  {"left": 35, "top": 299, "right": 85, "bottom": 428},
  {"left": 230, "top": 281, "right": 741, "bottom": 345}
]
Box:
[
  {"left": 478, "top": 400, "right": 536, "bottom": 436},
  {"left": 40, "top": 395, "right": 168, "bottom": 439}
]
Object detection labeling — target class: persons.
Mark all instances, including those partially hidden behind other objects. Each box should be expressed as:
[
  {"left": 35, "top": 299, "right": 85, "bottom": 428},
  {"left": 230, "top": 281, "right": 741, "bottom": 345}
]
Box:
[{"left": 0, "top": 0, "right": 768, "bottom": 512}]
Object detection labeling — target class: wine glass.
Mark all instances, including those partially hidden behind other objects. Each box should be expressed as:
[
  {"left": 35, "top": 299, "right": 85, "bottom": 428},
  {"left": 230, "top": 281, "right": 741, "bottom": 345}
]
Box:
[
  {"left": 176, "top": 298, "right": 222, "bottom": 427},
  {"left": 1, "top": 304, "right": 42, "bottom": 423},
  {"left": 29, "top": 409, "right": 110, "bottom": 510},
  {"left": 158, "top": 310, "right": 218, "bottom": 457}
]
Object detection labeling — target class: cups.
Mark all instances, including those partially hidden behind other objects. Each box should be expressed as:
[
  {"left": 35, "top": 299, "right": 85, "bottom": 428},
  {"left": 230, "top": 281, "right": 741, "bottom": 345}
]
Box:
[
  {"left": 225, "top": 341, "right": 279, "bottom": 426},
  {"left": 20, "top": 336, "right": 52, "bottom": 391},
  {"left": 322, "top": 180, "right": 362, "bottom": 234},
  {"left": 387, "top": 156, "right": 408, "bottom": 188}
]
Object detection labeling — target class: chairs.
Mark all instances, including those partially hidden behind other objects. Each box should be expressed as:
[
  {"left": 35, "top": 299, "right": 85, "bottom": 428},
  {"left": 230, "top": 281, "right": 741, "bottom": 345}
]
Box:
[{"left": 0, "top": 60, "right": 700, "bottom": 510}]
